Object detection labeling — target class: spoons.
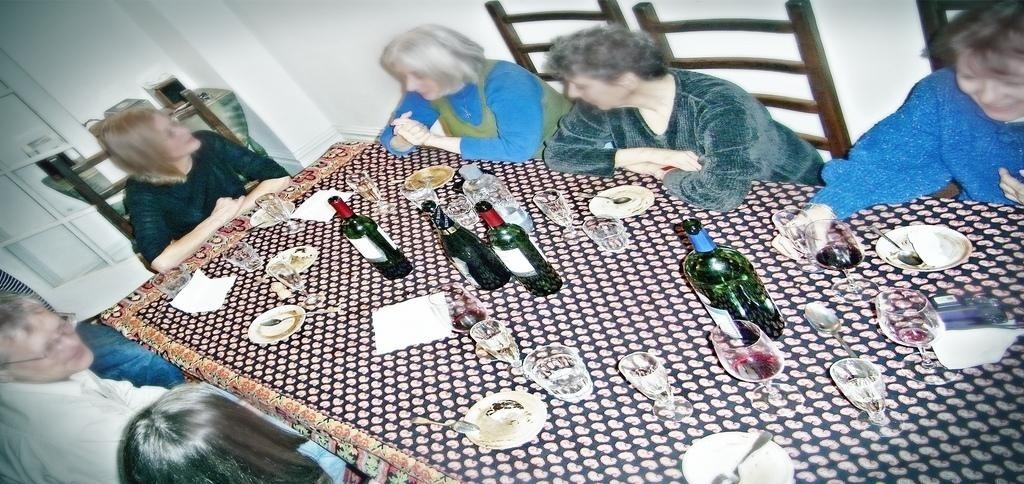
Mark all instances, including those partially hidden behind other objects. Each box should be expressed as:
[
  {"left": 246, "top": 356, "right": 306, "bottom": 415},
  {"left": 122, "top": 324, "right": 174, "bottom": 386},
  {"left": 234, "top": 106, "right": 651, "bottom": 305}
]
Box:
[
  {"left": 579, "top": 193, "right": 632, "bottom": 204},
  {"left": 411, "top": 416, "right": 480, "bottom": 435},
  {"left": 804, "top": 299, "right": 874, "bottom": 386},
  {"left": 867, "top": 225, "right": 924, "bottom": 266},
  {"left": 709, "top": 431, "right": 773, "bottom": 484},
  {"left": 260, "top": 307, "right": 338, "bottom": 326}
]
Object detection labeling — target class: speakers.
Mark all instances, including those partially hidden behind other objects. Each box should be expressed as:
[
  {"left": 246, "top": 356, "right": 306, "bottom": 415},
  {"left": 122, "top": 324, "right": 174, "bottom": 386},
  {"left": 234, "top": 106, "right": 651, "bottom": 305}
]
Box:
[
  {"left": 142, "top": 71, "right": 189, "bottom": 108},
  {"left": 36, "top": 147, "right": 76, "bottom": 182}
]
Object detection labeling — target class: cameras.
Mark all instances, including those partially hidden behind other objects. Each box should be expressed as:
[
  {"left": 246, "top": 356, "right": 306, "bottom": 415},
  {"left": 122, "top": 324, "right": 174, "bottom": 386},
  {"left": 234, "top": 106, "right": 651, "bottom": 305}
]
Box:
[{"left": 930, "top": 291, "right": 1006, "bottom": 325}]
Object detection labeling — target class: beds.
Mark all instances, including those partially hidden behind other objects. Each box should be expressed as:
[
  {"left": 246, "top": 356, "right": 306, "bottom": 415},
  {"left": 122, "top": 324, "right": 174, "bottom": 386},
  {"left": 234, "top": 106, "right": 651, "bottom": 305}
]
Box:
[{"left": 100, "top": 140, "right": 1024, "bottom": 484}]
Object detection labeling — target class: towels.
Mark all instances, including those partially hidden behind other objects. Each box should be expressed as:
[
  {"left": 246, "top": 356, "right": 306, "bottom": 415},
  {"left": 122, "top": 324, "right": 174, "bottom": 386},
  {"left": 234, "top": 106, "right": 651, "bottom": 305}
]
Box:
[
  {"left": 924, "top": 311, "right": 1024, "bottom": 369},
  {"left": 290, "top": 188, "right": 353, "bottom": 223},
  {"left": 169, "top": 270, "right": 237, "bottom": 312},
  {"left": 374, "top": 292, "right": 452, "bottom": 356}
]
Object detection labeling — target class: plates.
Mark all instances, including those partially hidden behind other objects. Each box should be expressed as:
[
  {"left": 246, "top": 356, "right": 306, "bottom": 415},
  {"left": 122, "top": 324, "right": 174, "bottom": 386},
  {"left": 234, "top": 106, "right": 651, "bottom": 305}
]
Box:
[
  {"left": 249, "top": 200, "right": 295, "bottom": 229},
  {"left": 874, "top": 224, "right": 973, "bottom": 273},
  {"left": 244, "top": 304, "right": 307, "bottom": 347},
  {"left": 588, "top": 186, "right": 656, "bottom": 217},
  {"left": 464, "top": 390, "right": 549, "bottom": 448},
  {"left": 681, "top": 431, "right": 804, "bottom": 484},
  {"left": 263, "top": 244, "right": 320, "bottom": 279},
  {"left": 403, "top": 164, "right": 456, "bottom": 191}
]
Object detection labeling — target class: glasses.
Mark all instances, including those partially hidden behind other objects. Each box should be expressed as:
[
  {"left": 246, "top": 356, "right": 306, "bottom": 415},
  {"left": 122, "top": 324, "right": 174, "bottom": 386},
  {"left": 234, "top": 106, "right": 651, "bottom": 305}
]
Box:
[{"left": 2, "top": 315, "right": 76, "bottom": 366}]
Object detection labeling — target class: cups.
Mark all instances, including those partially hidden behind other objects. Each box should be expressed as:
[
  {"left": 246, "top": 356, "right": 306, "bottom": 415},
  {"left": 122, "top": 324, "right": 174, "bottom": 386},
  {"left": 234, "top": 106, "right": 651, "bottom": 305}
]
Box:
[
  {"left": 525, "top": 344, "right": 594, "bottom": 404},
  {"left": 400, "top": 178, "right": 438, "bottom": 216},
  {"left": 150, "top": 265, "right": 193, "bottom": 299},
  {"left": 441, "top": 192, "right": 478, "bottom": 233},
  {"left": 223, "top": 241, "right": 264, "bottom": 273},
  {"left": 583, "top": 213, "right": 630, "bottom": 254}
]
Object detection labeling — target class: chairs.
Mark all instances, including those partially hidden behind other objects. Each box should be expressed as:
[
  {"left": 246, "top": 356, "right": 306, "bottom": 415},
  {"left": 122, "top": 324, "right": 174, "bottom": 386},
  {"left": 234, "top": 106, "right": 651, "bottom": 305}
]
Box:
[
  {"left": 485, "top": 0, "right": 631, "bottom": 82},
  {"left": 632, "top": 0, "right": 851, "bottom": 161},
  {"left": 916, "top": 0, "right": 977, "bottom": 72},
  {"left": 46, "top": 90, "right": 258, "bottom": 274}
]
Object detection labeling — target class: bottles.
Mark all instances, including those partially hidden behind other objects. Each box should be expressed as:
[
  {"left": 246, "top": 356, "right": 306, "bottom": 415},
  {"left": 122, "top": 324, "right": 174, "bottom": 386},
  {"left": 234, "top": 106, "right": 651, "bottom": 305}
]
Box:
[
  {"left": 420, "top": 202, "right": 513, "bottom": 289},
  {"left": 328, "top": 195, "right": 414, "bottom": 281},
  {"left": 474, "top": 201, "right": 564, "bottom": 297},
  {"left": 457, "top": 164, "right": 534, "bottom": 238},
  {"left": 680, "top": 218, "right": 783, "bottom": 350}
]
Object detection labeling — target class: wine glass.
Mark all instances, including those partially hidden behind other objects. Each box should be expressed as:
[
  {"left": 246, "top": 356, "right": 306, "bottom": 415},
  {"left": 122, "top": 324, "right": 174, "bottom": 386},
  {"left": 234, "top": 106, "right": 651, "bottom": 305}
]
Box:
[
  {"left": 802, "top": 216, "right": 881, "bottom": 304},
  {"left": 825, "top": 357, "right": 903, "bottom": 437},
  {"left": 269, "top": 261, "right": 320, "bottom": 305},
  {"left": 771, "top": 209, "right": 820, "bottom": 274},
  {"left": 878, "top": 292, "right": 959, "bottom": 385},
  {"left": 470, "top": 320, "right": 526, "bottom": 387},
  {"left": 712, "top": 322, "right": 803, "bottom": 417},
  {"left": 345, "top": 170, "right": 397, "bottom": 215},
  {"left": 428, "top": 287, "right": 487, "bottom": 367},
  {"left": 254, "top": 193, "right": 300, "bottom": 233},
  {"left": 533, "top": 189, "right": 579, "bottom": 243},
  {"left": 618, "top": 352, "right": 693, "bottom": 423}
]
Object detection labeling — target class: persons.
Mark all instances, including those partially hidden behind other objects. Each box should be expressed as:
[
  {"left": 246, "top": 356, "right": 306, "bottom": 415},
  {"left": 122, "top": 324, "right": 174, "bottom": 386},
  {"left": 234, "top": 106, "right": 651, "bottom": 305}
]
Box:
[
  {"left": 538, "top": 21, "right": 827, "bottom": 215},
  {"left": 0, "top": 290, "right": 171, "bottom": 482},
  {"left": 94, "top": 103, "right": 298, "bottom": 274},
  {"left": 783, "top": 0, "right": 1023, "bottom": 250},
  {"left": 377, "top": 23, "right": 573, "bottom": 164},
  {"left": 114, "top": 386, "right": 351, "bottom": 482}
]
